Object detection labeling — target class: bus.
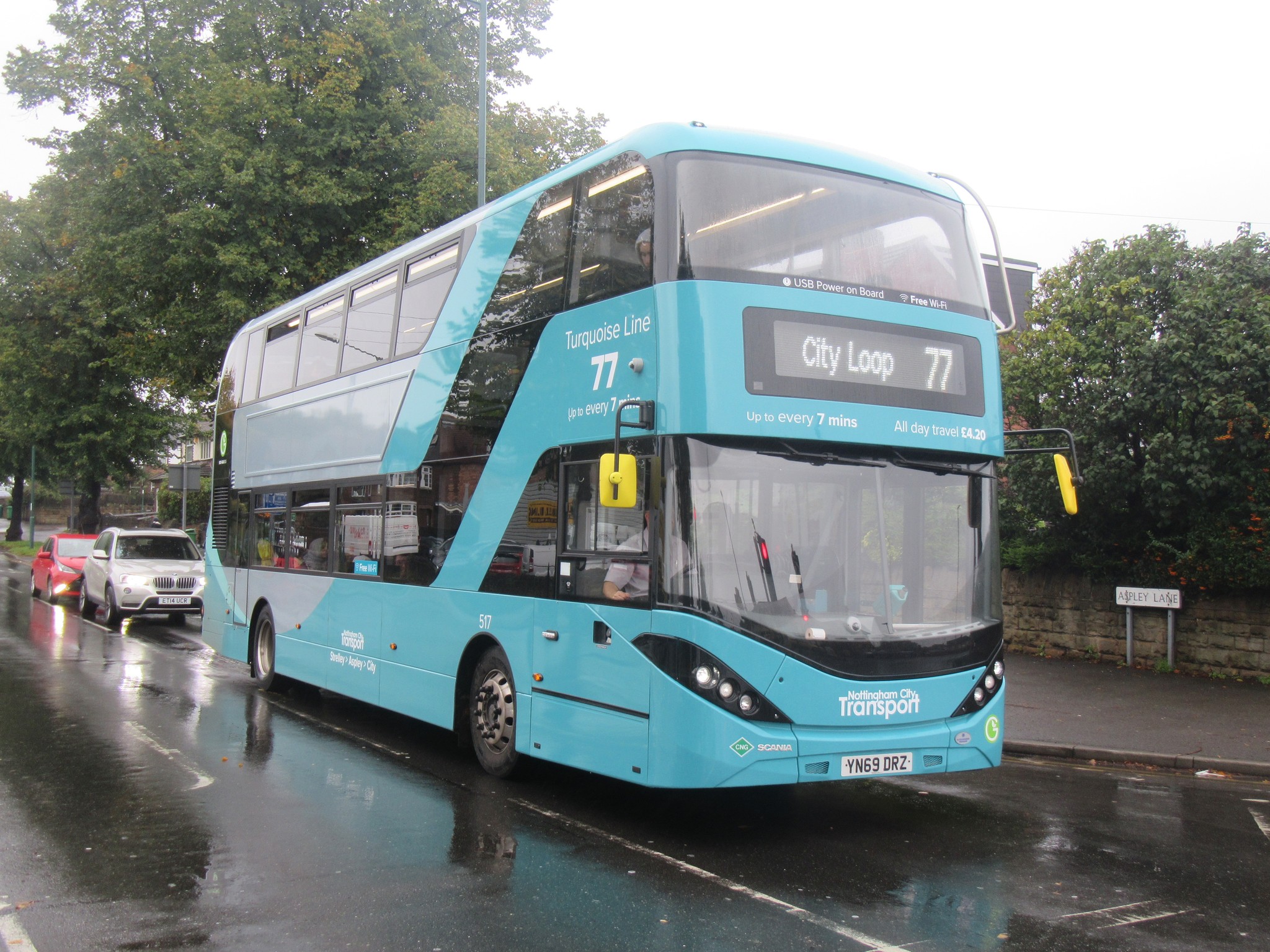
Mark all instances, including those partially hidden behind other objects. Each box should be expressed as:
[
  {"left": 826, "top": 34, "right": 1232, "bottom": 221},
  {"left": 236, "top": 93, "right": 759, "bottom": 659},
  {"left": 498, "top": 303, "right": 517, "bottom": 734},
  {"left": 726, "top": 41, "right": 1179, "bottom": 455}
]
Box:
[{"left": 200, "top": 124, "right": 1086, "bottom": 794}]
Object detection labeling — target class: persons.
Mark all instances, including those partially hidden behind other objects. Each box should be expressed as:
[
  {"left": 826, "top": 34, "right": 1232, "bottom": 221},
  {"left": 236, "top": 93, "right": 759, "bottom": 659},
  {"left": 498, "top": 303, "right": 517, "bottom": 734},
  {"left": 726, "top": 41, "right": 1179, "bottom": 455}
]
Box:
[
  {"left": 300, "top": 538, "right": 329, "bottom": 572},
  {"left": 635, "top": 228, "right": 651, "bottom": 272},
  {"left": 124, "top": 537, "right": 137, "bottom": 557},
  {"left": 151, "top": 517, "right": 162, "bottom": 529},
  {"left": 603, "top": 498, "right": 698, "bottom": 600},
  {"left": 393, "top": 553, "right": 412, "bottom": 585}
]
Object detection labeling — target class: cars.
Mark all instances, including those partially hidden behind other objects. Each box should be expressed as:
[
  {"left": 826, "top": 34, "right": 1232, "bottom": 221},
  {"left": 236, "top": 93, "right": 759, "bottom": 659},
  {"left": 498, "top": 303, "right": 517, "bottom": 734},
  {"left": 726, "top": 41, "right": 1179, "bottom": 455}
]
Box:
[
  {"left": 78, "top": 527, "right": 205, "bottom": 624},
  {"left": 29, "top": 533, "right": 104, "bottom": 604}
]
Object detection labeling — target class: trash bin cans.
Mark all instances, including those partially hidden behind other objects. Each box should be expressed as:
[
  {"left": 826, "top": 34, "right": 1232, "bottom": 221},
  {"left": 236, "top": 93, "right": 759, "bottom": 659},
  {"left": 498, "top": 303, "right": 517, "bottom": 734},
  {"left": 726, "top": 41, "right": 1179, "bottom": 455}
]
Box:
[
  {"left": 6, "top": 506, "right": 13, "bottom": 520},
  {"left": 0, "top": 505, "right": 3, "bottom": 518},
  {"left": 186, "top": 528, "right": 197, "bottom": 545}
]
